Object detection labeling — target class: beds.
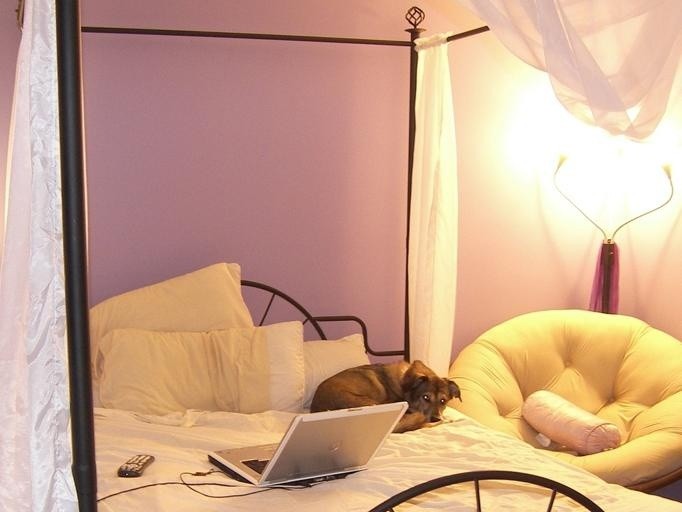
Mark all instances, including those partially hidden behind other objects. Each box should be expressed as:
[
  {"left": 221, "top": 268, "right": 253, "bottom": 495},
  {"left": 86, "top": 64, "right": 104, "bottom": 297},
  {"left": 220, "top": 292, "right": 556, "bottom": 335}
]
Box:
[{"left": 15, "top": 0, "right": 680, "bottom": 512}]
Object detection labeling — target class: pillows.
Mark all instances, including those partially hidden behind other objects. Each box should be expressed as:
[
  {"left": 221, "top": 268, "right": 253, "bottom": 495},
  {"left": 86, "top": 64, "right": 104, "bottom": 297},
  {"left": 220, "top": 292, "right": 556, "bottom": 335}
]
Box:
[
  {"left": 97, "top": 317, "right": 308, "bottom": 415},
  {"left": 521, "top": 385, "right": 621, "bottom": 458},
  {"left": 305, "top": 327, "right": 375, "bottom": 394},
  {"left": 89, "top": 253, "right": 255, "bottom": 363}
]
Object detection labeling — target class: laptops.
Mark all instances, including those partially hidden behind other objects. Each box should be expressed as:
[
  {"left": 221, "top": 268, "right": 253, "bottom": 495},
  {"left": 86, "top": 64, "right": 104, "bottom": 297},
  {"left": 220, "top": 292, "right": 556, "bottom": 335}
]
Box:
[{"left": 208, "top": 401, "right": 409, "bottom": 487}]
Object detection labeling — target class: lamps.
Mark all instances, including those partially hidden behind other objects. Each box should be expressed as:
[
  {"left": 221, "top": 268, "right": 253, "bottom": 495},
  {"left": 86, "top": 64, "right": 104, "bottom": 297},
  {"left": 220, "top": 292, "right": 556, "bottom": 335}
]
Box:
[{"left": 537, "top": 134, "right": 678, "bottom": 318}]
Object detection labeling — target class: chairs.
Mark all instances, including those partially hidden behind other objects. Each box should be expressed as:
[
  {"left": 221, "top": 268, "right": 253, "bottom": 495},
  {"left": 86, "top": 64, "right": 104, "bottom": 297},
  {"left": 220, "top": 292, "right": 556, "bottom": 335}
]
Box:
[{"left": 449, "top": 306, "right": 682, "bottom": 498}]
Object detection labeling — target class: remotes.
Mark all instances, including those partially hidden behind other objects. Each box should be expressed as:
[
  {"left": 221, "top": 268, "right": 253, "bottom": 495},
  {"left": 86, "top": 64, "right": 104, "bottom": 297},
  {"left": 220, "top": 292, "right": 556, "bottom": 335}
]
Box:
[{"left": 118, "top": 455, "right": 155, "bottom": 477}]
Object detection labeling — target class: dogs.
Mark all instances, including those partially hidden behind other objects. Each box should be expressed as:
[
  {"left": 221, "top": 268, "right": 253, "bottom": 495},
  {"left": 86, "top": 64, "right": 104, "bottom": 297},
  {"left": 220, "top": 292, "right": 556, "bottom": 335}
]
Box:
[{"left": 310, "top": 358, "right": 464, "bottom": 434}]
